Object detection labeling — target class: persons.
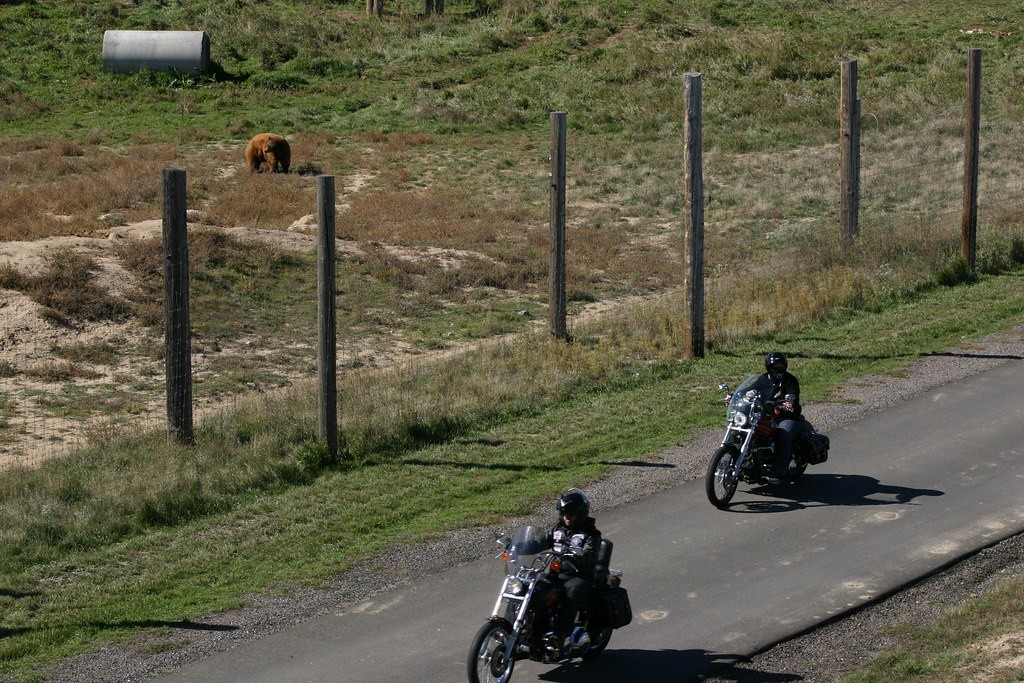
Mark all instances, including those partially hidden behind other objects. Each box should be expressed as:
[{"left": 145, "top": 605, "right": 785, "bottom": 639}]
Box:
[
  {"left": 503, "top": 489, "right": 602, "bottom": 652},
  {"left": 723, "top": 351, "right": 803, "bottom": 479}
]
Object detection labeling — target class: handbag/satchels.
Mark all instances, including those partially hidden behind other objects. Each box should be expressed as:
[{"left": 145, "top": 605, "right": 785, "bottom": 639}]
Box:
[{"left": 803, "top": 431, "right": 831, "bottom": 465}]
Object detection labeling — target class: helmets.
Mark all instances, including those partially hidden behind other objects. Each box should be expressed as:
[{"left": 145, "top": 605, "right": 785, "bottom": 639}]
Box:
[
  {"left": 556, "top": 493, "right": 591, "bottom": 531},
  {"left": 765, "top": 351, "right": 788, "bottom": 377}
]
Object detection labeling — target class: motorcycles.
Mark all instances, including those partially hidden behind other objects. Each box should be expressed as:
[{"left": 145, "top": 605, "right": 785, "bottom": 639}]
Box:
[
  {"left": 467, "top": 525, "right": 633, "bottom": 683},
  {"left": 705, "top": 383, "right": 829, "bottom": 509}
]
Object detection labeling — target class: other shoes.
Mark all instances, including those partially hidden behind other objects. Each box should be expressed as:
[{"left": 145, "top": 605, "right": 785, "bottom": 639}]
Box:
[
  {"left": 549, "top": 634, "right": 564, "bottom": 651},
  {"left": 766, "top": 467, "right": 788, "bottom": 484}
]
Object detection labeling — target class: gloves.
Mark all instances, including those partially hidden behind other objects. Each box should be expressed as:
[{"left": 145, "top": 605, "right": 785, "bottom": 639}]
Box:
[{"left": 559, "top": 552, "right": 575, "bottom": 570}]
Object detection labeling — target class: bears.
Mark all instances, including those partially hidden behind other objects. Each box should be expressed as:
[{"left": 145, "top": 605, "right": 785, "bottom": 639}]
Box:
[{"left": 246, "top": 133, "right": 290, "bottom": 174}]
